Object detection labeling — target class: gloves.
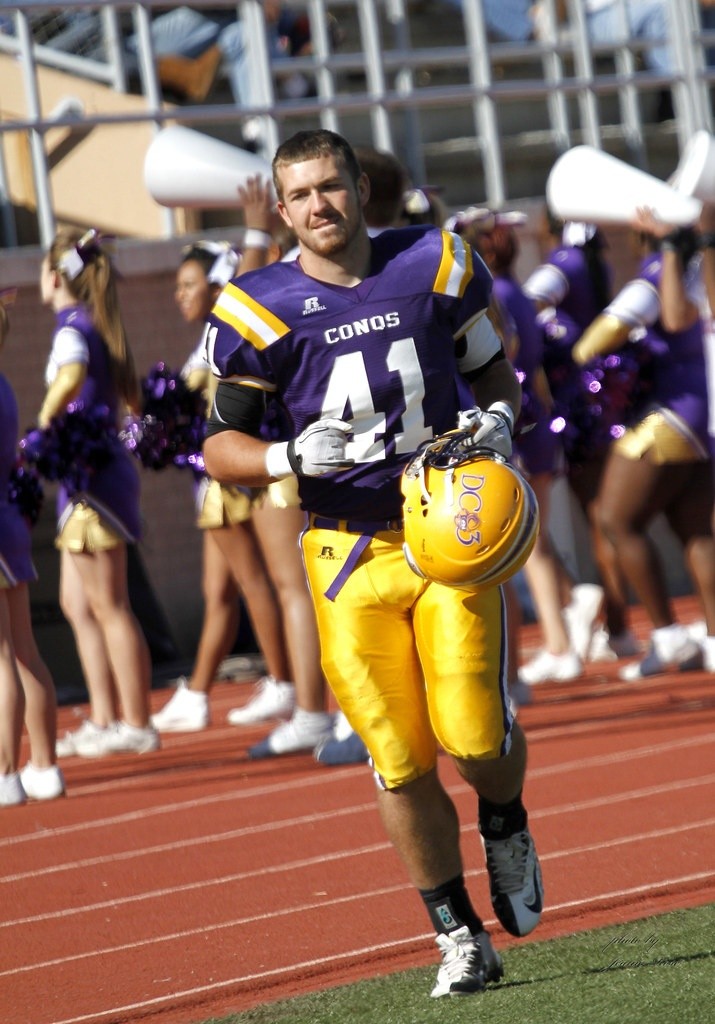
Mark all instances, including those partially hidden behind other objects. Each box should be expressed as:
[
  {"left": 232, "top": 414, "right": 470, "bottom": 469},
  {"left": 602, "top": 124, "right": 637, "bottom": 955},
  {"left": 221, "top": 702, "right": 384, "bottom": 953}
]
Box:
[
  {"left": 459, "top": 399, "right": 513, "bottom": 459},
  {"left": 266, "top": 418, "right": 356, "bottom": 480}
]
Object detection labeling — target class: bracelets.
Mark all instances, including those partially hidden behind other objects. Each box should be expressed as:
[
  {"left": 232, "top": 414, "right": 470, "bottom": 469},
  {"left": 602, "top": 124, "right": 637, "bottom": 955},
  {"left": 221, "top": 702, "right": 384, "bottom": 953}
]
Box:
[
  {"left": 242, "top": 228, "right": 273, "bottom": 249},
  {"left": 659, "top": 234, "right": 676, "bottom": 251},
  {"left": 700, "top": 232, "right": 715, "bottom": 249}
]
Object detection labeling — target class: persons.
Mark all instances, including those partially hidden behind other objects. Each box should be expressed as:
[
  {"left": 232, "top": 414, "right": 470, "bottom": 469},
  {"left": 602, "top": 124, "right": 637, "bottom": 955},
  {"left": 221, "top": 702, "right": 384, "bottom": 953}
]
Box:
[
  {"left": 446, "top": 201, "right": 715, "bottom": 687},
  {"left": 217, "top": 0, "right": 344, "bottom": 98},
  {"left": 151, "top": 146, "right": 449, "bottom": 764},
  {"left": 220, "top": 132, "right": 558, "bottom": 1000},
  {"left": 459, "top": 0, "right": 715, "bottom": 122},
  {"left": 0, "top": 305, "right": 65, "bottom": 806},
  {"left": 39, "top": 222, "right": 160, "bottom": 762}
]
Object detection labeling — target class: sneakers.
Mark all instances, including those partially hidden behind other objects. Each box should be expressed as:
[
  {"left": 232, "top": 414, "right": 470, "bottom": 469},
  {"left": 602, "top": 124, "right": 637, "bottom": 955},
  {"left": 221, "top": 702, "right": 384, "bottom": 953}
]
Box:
[
  {"left": 249, "top": 718, "right": 331, "bottom": 761},
  {"left": 518, "top": 651, "right": 585, "bottom": 682},
  {"left": 617, "top": 621, "right": 702, "bottom": 680},
  {"left": 316, "top": 718, "right": 369, "bottom": 765},
  {"left": 0, "top": 771, "right": 27, "bottom": 805},
  {"left": 223, "top": 675, "right": 293, "bottom": 724},
  {"left": 562, "top": 583, "right": 603, "bottom": 664},
  {"left": 19, "top": 761, "right": 64, "bottom": 798},
  {"left": 430, "top": 926, "right": 505, "bottom": 1000},
  {"left": 689, "top": 624, "right": 715, "bottom": 672},
  {"left": 78, "top": 721, "right": 159, "bottom": 757},
  {"left": 149, "top": 677, "right": 210, "bottom": 731},
  {"left": 481, "top": 826, "right": 547, "bottom": 938},
  {"left": 56, "top": 722, "right": 111, "bottom": 756}
]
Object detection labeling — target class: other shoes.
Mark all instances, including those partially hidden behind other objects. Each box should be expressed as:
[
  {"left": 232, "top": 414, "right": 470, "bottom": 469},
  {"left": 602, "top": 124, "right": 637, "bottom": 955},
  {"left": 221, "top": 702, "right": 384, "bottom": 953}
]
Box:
[{"left": 591, "top": 630, "right": 619, "bottom": 665}]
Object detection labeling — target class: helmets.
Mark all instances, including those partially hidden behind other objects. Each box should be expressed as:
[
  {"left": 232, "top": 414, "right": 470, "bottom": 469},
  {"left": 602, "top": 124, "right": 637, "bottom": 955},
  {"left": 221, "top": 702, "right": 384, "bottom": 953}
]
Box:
[{"left": 400, "top": 430, "right": 540, "bottom": 593}]
